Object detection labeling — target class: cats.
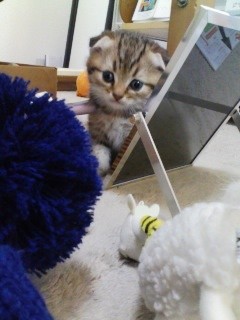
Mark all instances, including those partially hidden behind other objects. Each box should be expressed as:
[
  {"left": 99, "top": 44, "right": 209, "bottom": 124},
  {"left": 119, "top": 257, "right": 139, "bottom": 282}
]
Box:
[{"left": 87, "top": 28, "right": 169, "bottom": 176}]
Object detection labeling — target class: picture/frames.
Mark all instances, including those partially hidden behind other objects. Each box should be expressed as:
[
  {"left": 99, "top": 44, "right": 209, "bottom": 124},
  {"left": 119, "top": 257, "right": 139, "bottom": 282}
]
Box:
[{"left": 102, "top": 5, "right": 240, "bottom": 191}]
